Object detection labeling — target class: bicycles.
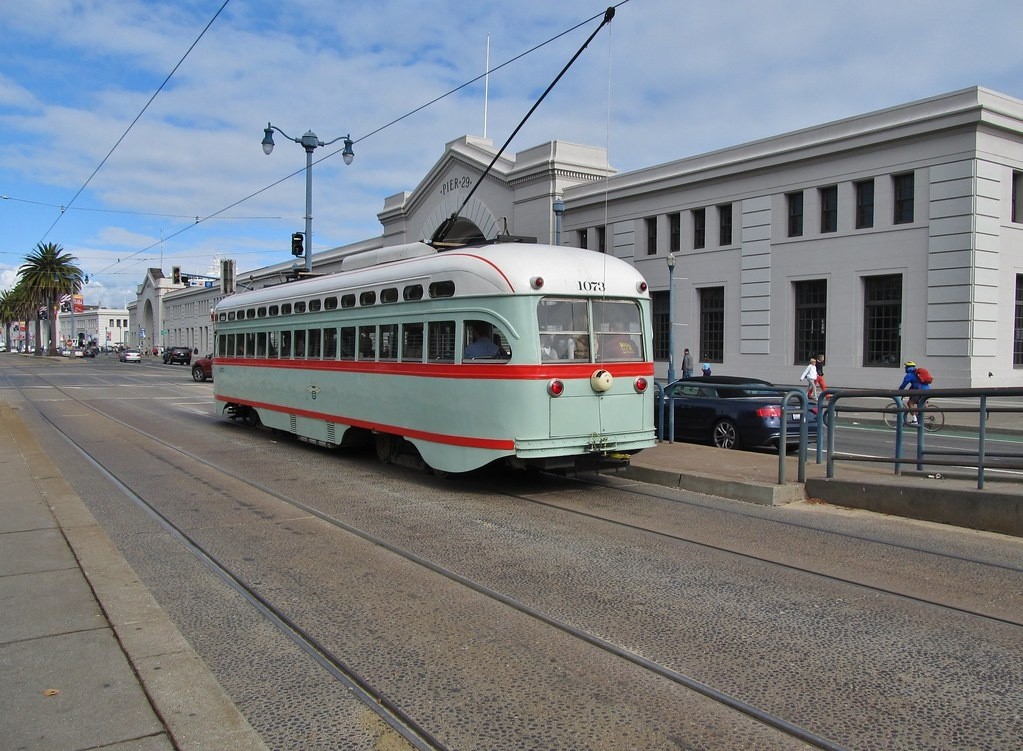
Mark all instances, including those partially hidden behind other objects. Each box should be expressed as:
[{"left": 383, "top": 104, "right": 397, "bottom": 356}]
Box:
[{"left": 883, "top": 396, "right": 945, "bottom": 432}]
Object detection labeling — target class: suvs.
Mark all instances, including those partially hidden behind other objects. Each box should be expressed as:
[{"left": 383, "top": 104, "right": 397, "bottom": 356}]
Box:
[{"left": 167, "top": 347, "right": 192, "bottom": 364}]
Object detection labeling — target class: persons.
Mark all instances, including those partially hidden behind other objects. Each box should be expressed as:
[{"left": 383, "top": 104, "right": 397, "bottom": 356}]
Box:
[
  {"left": 464, "top": 323, "right": 500, "bottom": 359},
  {"left": 898, "top": 361, "right": 930, "bottom": 424},
  {"left": 800, "top": 358, "right": 819, "bottom": 401},
  {"left": 189, "top": 346, "right": 193, "bottom": 358},
  {"left": 98, "top": 346, "right": 129, "bottom": 354},
  {"left": 807, "top": 355, "right": 832, "bottom": 400},
  {"left": 257, "top": 333, "right": 275, "bottom": 356},
  {"left": 567, "top": 313, "right": 597, "bottom": 360},
  {"left": 493, "top": 334, "right": 501, "bottom": 346},
  {"left": 702, "top": 358, "right": 711, "bottom": 376},
  {"left": 540, "top": 328, "right": 558, "bottom": 359},
  {"left": 194, "top": 346, "right": 198, "bottom": 359},
  {"left": 599, "top": 321, "right": 639, "bottom": 357},
  {"left": 681, "top": 349, "right": 693, "bottom": 378},
  {"left": 145, "top": 347, "right": 164, "bottom": 356}
]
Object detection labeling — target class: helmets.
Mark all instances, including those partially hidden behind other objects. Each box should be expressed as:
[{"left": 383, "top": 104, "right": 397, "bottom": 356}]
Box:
[{"left": 904, "top": 361, "right": 916, "bottom": 367}]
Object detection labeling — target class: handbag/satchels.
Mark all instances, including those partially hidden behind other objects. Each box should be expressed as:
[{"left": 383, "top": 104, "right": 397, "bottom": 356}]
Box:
[{"left": 702, "top": 368, "right": 711, "bottom": 376}]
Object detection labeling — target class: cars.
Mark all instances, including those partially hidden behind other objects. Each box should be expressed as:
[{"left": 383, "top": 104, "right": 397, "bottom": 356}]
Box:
[
  {"left": 74, "top": 349, "right": 83, "bottom": 357},
  {"left": 191, "top": 352, "right": 213, "bottom": 382},
  {"left": 120, "top": 349, "right": 141, "bottom": 363},
  {"left": 654, "top": 375, "right": 818, "bottom": 451},
  {"left": 83, "top": 349, "right": 95, "bottom": 358},
  {"left": 56, "top": 347, "right": 71, "bottom": 356}
]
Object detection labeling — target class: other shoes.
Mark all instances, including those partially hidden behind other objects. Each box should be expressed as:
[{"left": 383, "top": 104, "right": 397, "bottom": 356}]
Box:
[
  {"left": 827, "top": 396, "right": 832, "bottom": 400},
  {"left": 808, "top": 397, "right": 814, "bottom": 400},
  {"left": 908, "top": 420, "right": 918, "bottom": 425},
  {"left": 814, "top": 398, "right": 818, "bottom": 401}
]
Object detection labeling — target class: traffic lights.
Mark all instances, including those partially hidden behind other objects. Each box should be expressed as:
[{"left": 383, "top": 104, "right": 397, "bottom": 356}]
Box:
[
  {"left": 172, "top": 266, "right": 181, "bottom": 284},
  {"left": 291, "top": 234, "right": 304, "bottom": 256},
  {"left": 181, "top": 276, "right": 188, "bottom": 283},
  {"left": 220, "top": 257, "right": 236, "bottom": 295}
]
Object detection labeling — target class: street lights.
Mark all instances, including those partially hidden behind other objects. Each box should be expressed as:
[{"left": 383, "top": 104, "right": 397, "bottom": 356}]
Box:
[
  {"left": 261, "top": 123, "right": 355, "bottom": 272},
  {"left": 62, "top": 274, "right": 89, "bottom": 347},
  {"left": 664, "top": 252, "right": 677, "bottom": 443}
]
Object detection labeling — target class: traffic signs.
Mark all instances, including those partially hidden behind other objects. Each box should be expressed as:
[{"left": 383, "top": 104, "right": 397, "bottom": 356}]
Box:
[{"left": 189, "top": 280, "right": 213, "bottom": 288}]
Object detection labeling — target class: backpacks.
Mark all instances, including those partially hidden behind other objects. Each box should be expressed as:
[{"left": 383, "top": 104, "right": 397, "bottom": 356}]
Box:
[{"left": 913, "top": 368, "right": 933, "bottom": 384}]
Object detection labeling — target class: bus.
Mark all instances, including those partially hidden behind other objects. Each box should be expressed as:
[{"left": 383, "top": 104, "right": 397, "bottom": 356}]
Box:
[{"left": 212, "top": 239, "right": 658, "bottom": 490}]
